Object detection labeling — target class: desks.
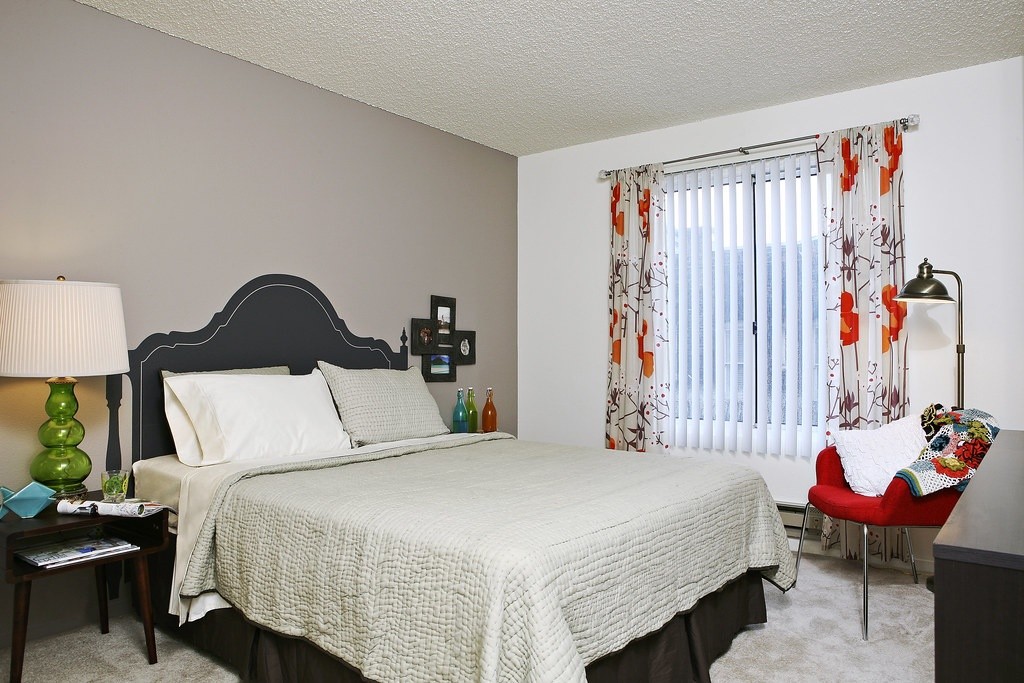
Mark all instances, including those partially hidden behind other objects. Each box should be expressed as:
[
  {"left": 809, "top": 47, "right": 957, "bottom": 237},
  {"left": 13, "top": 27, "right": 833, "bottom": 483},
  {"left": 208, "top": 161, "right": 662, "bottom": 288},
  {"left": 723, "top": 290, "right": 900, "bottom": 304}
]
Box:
[
  {"left": 931, "top": 430, "right": 1024, "bottom": 683},
  {"left": 0, "top": 490, "right": 169, "bottom": 683}
]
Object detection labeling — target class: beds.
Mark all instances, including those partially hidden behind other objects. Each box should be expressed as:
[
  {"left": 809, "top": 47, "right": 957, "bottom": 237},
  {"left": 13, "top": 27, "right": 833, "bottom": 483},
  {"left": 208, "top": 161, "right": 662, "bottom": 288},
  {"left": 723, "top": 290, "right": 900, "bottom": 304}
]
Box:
[{"left": 125, "top": 273, "right": 796, "bottom": 683}]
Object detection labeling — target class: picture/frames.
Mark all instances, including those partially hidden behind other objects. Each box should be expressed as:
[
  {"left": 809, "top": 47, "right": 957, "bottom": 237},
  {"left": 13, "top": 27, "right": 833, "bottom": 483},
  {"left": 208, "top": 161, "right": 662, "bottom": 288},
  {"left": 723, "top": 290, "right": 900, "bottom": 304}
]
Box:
[
  {"left": 453, "top": 330, "right": 476, "bottom": 366},
  {"left": 411, "top": 319, "right": 438, "bottom": 355},
  {"left": 430, "top": 295, "right": 456, "bottom": 344},
  {"left": 422, "top": 347, "right": 456, "bottom": 382}
]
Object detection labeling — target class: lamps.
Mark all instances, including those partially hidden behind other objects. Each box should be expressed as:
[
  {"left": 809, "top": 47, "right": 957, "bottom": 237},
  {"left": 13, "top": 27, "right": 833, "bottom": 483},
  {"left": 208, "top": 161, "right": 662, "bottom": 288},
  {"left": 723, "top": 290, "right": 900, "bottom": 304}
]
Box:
[
  {"left": 0, "top": 280, "right": 130, "bottom": 503},
  {"left": 893, "top": 258, "right": 965, "bottom": 409}
]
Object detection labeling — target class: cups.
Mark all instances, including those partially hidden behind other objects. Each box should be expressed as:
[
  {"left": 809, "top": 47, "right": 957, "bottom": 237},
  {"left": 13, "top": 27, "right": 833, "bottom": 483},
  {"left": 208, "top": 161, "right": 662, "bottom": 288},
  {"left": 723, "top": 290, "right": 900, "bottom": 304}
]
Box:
[{"left": 101, "top": 470, "right": 129, "bottom": 503}]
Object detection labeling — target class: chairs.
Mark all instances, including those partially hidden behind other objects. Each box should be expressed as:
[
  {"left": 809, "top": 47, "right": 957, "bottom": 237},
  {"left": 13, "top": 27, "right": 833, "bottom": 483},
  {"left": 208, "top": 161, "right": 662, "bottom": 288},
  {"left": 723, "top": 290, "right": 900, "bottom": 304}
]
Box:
[{"left": 792, "top": 404, "right": 1000, "bottom": 640}]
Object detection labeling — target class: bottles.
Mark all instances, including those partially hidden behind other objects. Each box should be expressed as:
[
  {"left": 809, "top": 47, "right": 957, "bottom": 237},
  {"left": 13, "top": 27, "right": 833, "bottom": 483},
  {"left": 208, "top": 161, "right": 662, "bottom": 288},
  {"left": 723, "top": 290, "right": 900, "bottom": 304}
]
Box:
[
  {"left": 482, "top": 388, "right": 497, "bottom": 433},
  {"left": 452, "top": 388, "right": 469, "bottom": 433},
  {"left": 465, "top": 387, "right": 478, "bottom": 433}
]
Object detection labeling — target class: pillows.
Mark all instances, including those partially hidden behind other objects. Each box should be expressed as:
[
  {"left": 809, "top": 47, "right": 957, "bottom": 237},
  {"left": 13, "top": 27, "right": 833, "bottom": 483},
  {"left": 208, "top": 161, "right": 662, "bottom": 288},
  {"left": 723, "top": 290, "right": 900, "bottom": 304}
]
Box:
[
  {"left": 163, "top": 368, "right": 351, "bottom": 466},
  {"left": 316, "top": 360, "right": 450, "bottom": 448}
]
